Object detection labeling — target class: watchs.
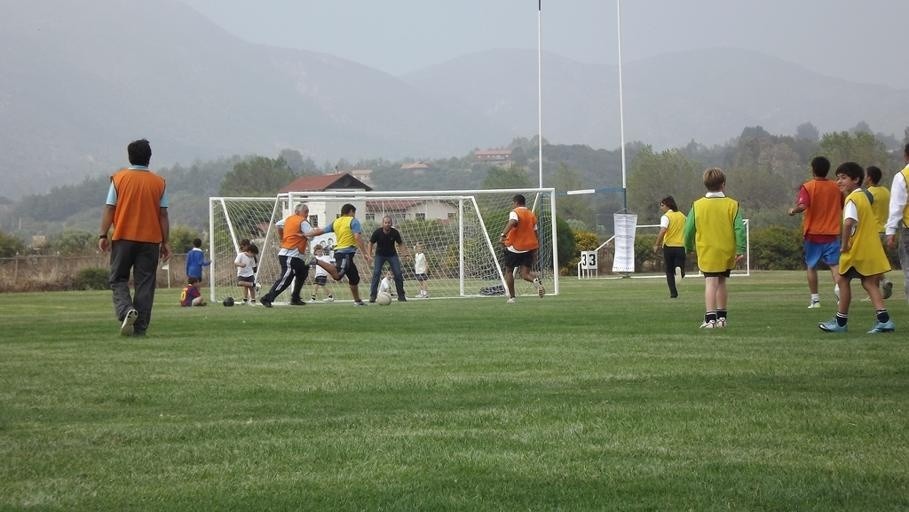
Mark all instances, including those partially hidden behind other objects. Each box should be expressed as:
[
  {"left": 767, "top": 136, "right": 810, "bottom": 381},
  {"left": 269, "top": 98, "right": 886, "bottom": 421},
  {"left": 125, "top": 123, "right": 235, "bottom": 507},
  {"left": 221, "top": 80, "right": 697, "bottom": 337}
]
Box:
[{"left": 501, "top": 232, "right": 506, "bottom": 237}]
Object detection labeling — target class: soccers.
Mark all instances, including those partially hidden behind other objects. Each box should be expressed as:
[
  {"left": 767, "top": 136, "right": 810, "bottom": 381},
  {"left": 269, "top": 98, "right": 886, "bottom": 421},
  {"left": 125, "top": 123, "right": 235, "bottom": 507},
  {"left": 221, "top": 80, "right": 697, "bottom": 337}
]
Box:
[
  {"left": 223, "top": 298, "right": 234, "bottom": 306},
  {"left": 378, "top": 293, "right": 390, "bottom": 305}
]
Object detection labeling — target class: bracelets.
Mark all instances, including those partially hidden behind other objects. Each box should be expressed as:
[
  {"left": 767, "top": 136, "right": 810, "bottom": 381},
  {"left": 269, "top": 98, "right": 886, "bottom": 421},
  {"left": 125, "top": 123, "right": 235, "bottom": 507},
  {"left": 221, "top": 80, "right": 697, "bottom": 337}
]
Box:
[
  {"left": 99, "top": 235, "right": 109, "bottom": 239},
  {"left": 791, "top": 209, "right": 795, "bottom": 215}
]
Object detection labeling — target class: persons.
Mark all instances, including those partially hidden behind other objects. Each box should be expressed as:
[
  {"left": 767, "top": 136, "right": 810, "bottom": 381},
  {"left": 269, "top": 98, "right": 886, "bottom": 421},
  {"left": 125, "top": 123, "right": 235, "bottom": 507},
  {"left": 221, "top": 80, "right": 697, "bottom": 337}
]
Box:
[
  {"left": 882, "top": 141, "right": 908, "bottom": 308},
  {"left": 97, "top": 135, "right": 172, "bottom": 341},
  {"left": 816, "top": 162, "right": 896, "bottom": 336},
  {"left": 786, "top": 155, "right": 846, "bottom": 310},
  {"left": 683, "top": 166, "right": 750, "bottom": 331},
  {"left": 179, "top": 202, "right": 431, "bottom": 308},
  {"left": 498, "top": 195, "right": 547, "bottom": 304},
  {"left": 857, "top": 165, "right": 894, "bottom": 304},
  {"left": 654, "top": 195, "right": 690, "bottom": 298}
]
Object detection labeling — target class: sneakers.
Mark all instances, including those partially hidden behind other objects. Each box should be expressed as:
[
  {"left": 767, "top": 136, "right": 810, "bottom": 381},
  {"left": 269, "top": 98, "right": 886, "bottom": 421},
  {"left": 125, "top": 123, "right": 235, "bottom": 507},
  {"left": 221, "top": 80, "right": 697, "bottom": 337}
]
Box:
[
  {"left": 881, "top": 278, "right": 893, "bottom": 300},
  {"left": 864, "top": 321, "right": 896, "bottom": 333},
  {"left": 699, "top": 320, "right": 716, "bottom": 328},
  {"left": 674, "top": 265, "right": 682, "bottom": 284},
  {"left": 120, "top": 305, "right": 140, "bottom": 336},
  {"left": 303, "top": 253, "right": 315, "bottom": 268},
  {"left": 716, "top": 317, "right": 727, "bottom": 329},
  {"left": 506, "top": 297, "right": 516, "bottom": 304},
  {"left": 806, "top": 299, "right": 821, "bottom": 308},
  {"left": 834, "top": 290, "right": 840, "bottom": 306},
  {"left": 533, "top": 277, "right": 546, "bottom": 299},
  {"left": 817, "top": 317, "right": 849, "bottom": 334},
  {"left": 240, "top": 291, "right": 430, "bottom": 307}
]
